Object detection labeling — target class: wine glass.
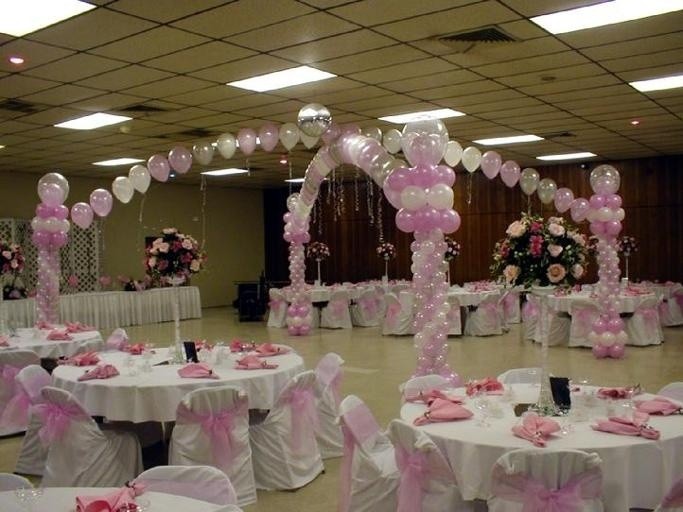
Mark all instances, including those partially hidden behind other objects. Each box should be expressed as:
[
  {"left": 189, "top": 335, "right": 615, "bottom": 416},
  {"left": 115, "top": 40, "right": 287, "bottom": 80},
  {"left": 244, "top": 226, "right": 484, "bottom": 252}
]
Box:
[
  {"left": 14, "top": 485, "right": 44, "bottom": 512},
  {"left": 473, "top": 389, "right": 495, "bottom": 428}
]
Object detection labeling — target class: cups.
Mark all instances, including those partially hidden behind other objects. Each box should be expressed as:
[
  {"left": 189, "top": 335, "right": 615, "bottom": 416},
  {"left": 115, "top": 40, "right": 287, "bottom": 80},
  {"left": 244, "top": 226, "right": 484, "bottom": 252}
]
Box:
[
  {"left": 557, "top": 405, "right": 577, "bottom": 436},
  {"left": 620, "top": 401, "right": 634, "bottom": 420}
]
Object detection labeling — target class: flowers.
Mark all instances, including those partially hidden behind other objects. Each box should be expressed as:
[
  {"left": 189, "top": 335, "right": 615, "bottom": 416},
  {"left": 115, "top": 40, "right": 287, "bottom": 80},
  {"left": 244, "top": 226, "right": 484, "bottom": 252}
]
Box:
[
  {"left": 0, "top": 240, "right": 26, "bottom": 275},
  {"left": 140, "top": 226, "right": 207, "bottom": 281},
  {"left": 444, "top": 236, "right": 460, "bottom": 262},
  {"left": 618, "top": 235, "right": 639, "bottom": 257},
  {"left": 305, "top": 241, "right": 330, "bottom": 261},
  {"left": 488, "top": 212, "right": 591, "bottom": 290},
  {"left": 375, "top": 243, "right": 397, "bottom": 262},
  {"left": 588, "top": 235, "right": 599, "bottom": 257}
]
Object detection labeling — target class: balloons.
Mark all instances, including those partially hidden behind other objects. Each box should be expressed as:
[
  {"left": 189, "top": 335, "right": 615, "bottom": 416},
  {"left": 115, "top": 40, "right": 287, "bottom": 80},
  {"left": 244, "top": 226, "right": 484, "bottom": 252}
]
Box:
[
  {"left": 282, "top": 116, "right": 462, "bottom": 390},
  {"left": 32, "top": 171, "right": 69, "bottom": 324},
  {"left": 518, "top": 168, "right": 539, "bottom": 195},
  {"left": 462, "top": 146, "right": 483, "bottom": 173},
  {"left": 537, "top": 177, "right": 557, "bottom": 204},
  {"left": 71, "top": 123, "right": 282, "bottom": 230},
  {"left": 554, "top": 187, "right": 574, "bottom": 213},
  {"left": 500, "top": 160, "right": 520, "bottom": 187},
  {"left": 583, "top": 163, "right": 627, "bottom": 360},
  {"left": 481, "top": 150, "right": 502, "bottom": 180},
  {"left": 570, "top": 196, "right": 589, "bottom": 223}
]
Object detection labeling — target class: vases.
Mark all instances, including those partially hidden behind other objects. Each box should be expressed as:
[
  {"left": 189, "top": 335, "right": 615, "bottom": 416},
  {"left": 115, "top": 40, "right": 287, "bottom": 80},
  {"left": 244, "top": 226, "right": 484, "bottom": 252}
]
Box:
[
  {"left": 316, "top": 258, "right": 321, "bottom": 285},
  {"left": 623, "top": 251, "right": 629, "bottom": 282},
  {"left": 384, "top": 256, "right": 388, "bottom": 281}
]
